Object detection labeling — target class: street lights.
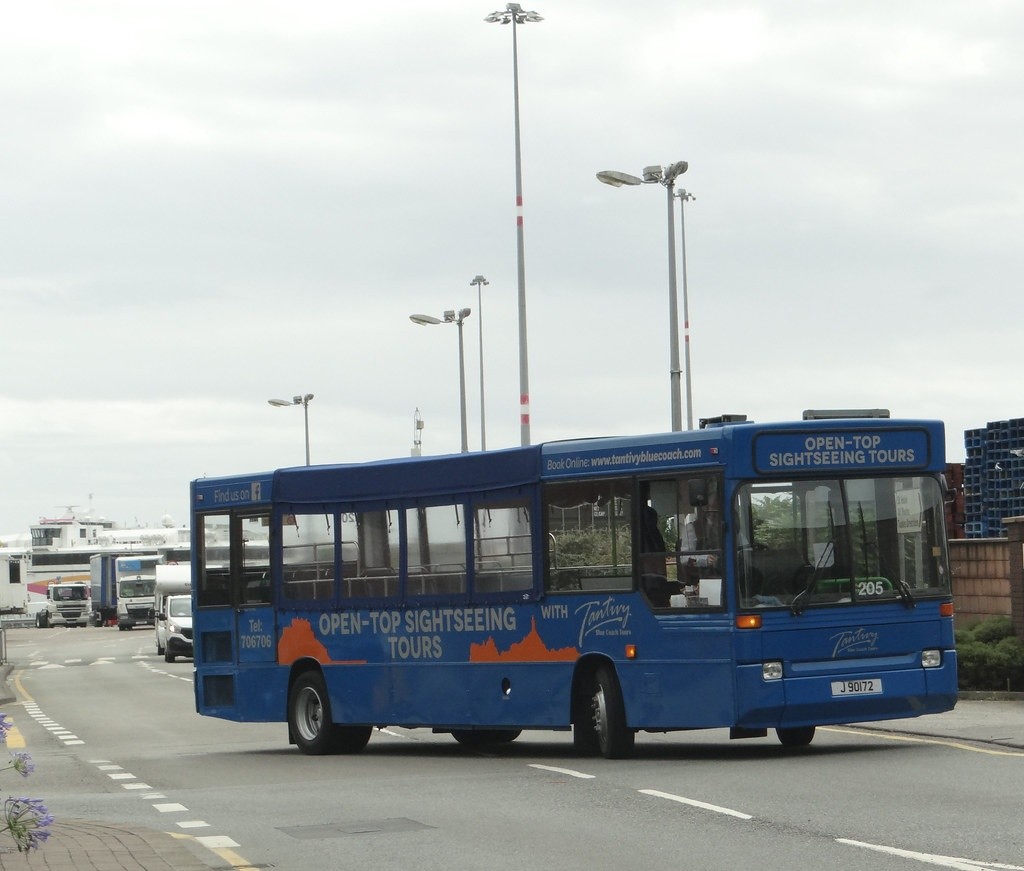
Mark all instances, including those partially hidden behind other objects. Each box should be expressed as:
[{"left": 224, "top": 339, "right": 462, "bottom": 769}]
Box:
[
  {"left": 596, "top": 157, "right": 698, "bottom": 435},
  {"left": 411, "top": 306, "right": 475, "bottom": 455},
  {"left": 268, "top": 393, "right": 314, "bottom": 467},
  {"left": 486, "top": 3, "right": 544, "bottom": 453},
  {"left": 470, "top": 274, "right": 490, "bottom": 453}
]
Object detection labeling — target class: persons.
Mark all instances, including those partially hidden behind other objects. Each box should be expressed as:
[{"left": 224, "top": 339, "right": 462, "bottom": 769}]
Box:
[{"left": 680, "top": 492, "right": 753, "bottom": 578}]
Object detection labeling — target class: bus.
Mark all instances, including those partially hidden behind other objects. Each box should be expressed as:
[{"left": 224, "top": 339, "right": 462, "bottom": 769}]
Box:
[{"left": 188, "top": 406, "right": 961, "bottom": 758}]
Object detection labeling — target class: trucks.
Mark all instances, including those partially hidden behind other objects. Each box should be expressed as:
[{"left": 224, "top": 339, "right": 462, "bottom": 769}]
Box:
[{"left": 36, "top": 553, "right": 223, "bottom": 663}]
